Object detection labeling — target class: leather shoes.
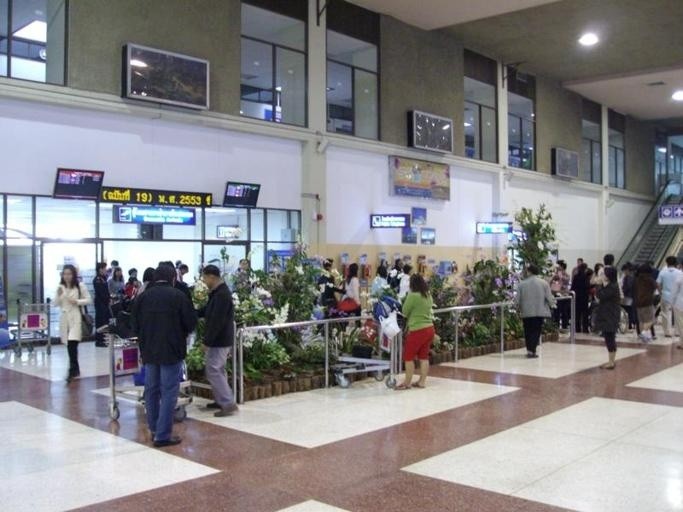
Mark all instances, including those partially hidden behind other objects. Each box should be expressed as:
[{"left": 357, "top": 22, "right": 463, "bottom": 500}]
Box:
[
  {"left": 153, "top": 439, "right": 181, "bottom": 447},
  {"left": 150, "top": 434, "right": 180, "bottom": 441}
]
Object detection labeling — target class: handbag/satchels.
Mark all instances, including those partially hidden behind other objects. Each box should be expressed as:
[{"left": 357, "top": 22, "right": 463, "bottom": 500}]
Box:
[
  {"left": 81, "top": 315, "right": 93, "bottom": 336},
  {"left": 337, "top": 298, "right": 358, "bottom": 311}
]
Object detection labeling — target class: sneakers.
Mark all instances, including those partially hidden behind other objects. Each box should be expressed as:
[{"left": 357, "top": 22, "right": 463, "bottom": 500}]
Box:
[
  {"left": 528, "top": 353, "right": 538, "bottom": 357},
  {"left": 207, "top": 402, "right": 220, "bottom": 408},
  {"left": 215, "top": 405, "right": 238, "bottom": 417}
]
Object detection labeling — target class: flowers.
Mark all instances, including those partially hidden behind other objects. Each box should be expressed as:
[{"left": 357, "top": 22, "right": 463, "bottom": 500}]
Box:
[
  {"left": 433, "top": 272, "right": 526, "bottom": 347},
  {"left": 187, "top": 232, "right": 341, "bottom": 376}
]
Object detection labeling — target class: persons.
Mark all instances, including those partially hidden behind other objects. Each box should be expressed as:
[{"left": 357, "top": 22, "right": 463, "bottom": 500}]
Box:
[
  {"left": 129, "top": 261, "right": 200, "bottom": 448},
  {"left": 316, "top": 258, "right": 419, "bottom": 333},
  {"left": 593, "top": 267, "right": 620, "bottom": 369},
  {"left": 192, "top": 264, "right": 239, "bottom": 417},
  {"left": 52, "top": 264, "right": 92, "bottom": 383},
  {"left": 234, "top": 259, "right": 251, "bottom": 294},
  {"left": 392, "top": 273, "right": 436, "bottom": 389},
  {"left": 551, "top": 255, "right": 682, "bottom": 353},
  {"left": 88, "top": 260, "right": 189, "bottom": 348},
  {"left": 515, "top": 264, "right": 558, "bottom": 358}
]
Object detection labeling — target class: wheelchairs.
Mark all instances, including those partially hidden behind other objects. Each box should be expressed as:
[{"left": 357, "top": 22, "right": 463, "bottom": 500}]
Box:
[{"left": 588, "top": 304, "right": 629, "bottom": 336}]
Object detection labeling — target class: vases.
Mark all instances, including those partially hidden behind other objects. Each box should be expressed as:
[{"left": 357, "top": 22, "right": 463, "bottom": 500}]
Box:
[
  {"left": 541, "top": 332, "right": 561, "bottom": 343},
  {"left": 228, "top": 375, "right": 325, "bottom": 406},
  {"left": 428, "top": 338, "right": 526, "bottom": 366},
  {"left": 191, "top": 378, "right": 213, "bottom": 400},
  {"left": 329, "top": 372, "right": 376, "bottom": 387}
]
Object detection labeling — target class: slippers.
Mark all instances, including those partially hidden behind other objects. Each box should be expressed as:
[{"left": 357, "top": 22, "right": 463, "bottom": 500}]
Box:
[
  {"left": 411, "top": 383, "right": 425, "bottom": 388},
  {"left": 395, "top": 384, "right": 411, "bottom": 390}
]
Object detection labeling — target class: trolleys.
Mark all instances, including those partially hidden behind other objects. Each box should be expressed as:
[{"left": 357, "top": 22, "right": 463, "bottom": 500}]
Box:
[
  {"left": 108, "top": 334, "right": 193, "bottom": 422},
  {"left": 331, "top": 337, "right": 396, "bottom": 389},
  {"left": 11, "top": 298, "right": 51, "bottom": 358}
]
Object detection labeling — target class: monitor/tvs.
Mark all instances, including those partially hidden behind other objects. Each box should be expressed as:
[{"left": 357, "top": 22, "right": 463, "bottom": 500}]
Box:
[
  {"left": 411, "top": 108, "right": 454, "bottom": 155},
  {"left": 223, "top": 181, "right": 261, "bottom": 208},
  {"left": 552, "top": 145, "right": 580, "bottom": 182},
  {"left": 54, "top": 168, "right": 105, "bottom": 201},
  {"left": 123, "top": 42, "right": 211, "bottom": 113}
]
